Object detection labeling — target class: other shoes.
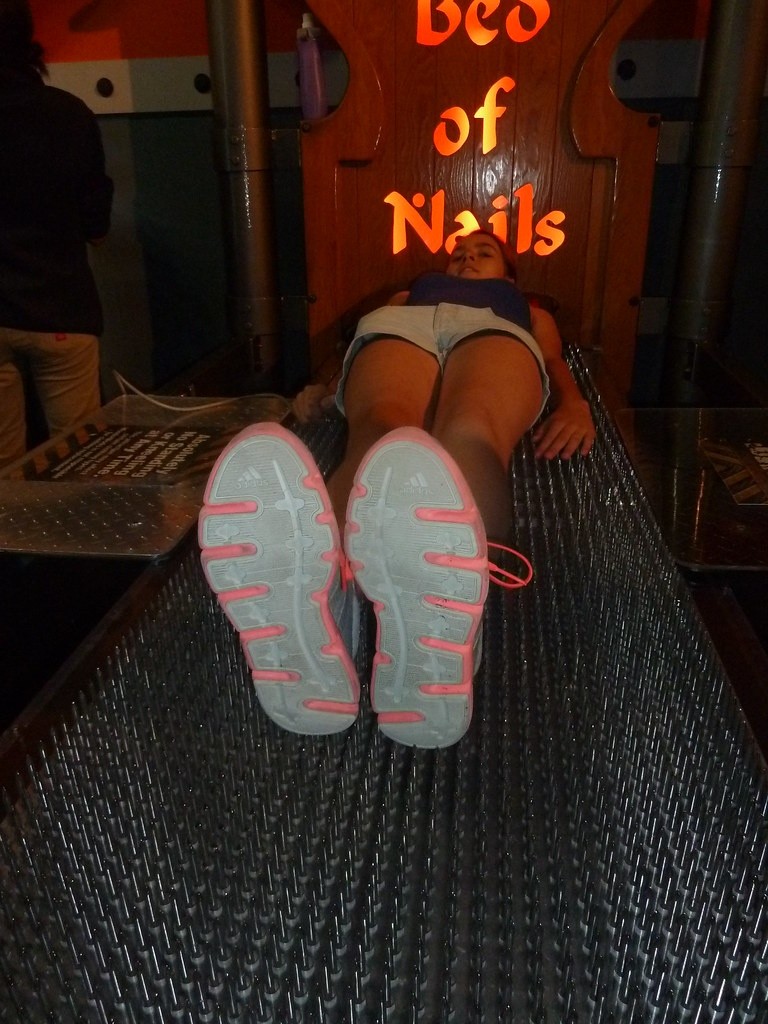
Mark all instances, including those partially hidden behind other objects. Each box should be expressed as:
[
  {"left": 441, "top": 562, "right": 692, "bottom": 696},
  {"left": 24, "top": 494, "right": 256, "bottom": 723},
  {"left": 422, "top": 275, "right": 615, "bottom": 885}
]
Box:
[
  {"left": 343, "top": 426, "right": 534, "bottom": 750},
  {"left": 199, "top": 422, "right": 362, "bottom": 736}
]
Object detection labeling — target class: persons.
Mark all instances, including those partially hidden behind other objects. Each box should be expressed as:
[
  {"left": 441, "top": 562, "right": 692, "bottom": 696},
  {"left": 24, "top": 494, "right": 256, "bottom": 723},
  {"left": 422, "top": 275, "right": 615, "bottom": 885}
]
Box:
[
  {"left": 0, "top": 0, "right": 117, "bottom": 471},
  {"left": 198, "top": 229, "right": 598, "bottom": 746}
]
